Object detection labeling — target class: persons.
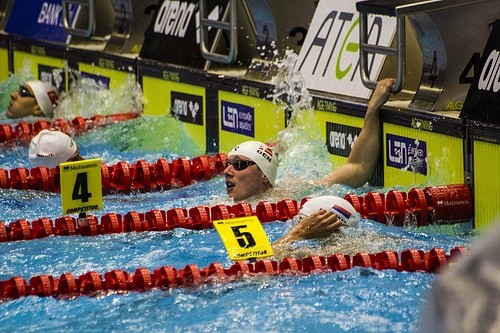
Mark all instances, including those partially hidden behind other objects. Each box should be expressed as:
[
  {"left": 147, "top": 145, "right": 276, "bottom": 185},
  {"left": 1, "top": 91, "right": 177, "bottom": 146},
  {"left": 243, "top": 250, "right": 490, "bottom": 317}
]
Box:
[
  {"left": 271, "top": 194, "right": 362, "bottom": 253},
  {"left": 5, "top": 81, "right": 59, "bottom": 119},
  {"left": 28, "top": 129, "right": 87, "bottom": 169},
  {"left": 223, "top": 78, "right": 397, "bottom": 201}
]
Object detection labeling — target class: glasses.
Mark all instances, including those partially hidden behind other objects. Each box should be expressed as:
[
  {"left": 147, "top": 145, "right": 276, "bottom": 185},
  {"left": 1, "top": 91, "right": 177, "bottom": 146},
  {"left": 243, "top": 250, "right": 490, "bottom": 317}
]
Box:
[
  {"left": 18, "top": 87, "right": 34, "bottom": 98},
  {"left": 73, "top": 154, "right": 80, "bottom": 161},
  {"left": 225, "top": 160, "right": 256, "bottom": 171}
]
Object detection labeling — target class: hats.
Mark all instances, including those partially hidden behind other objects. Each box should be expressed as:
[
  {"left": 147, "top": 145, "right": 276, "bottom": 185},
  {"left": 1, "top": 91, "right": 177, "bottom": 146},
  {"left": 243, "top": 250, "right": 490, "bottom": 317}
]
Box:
[
  {"left": 227, "top": 141, "right": 277, "bottom": 186},
  {"left": 27, "top": 81, "right": 60, "bottom": 119},
  {"left": 28, "top": 129, "right": 76, "bottom": 169},
  {"left": 298, "top": 195, "right": 359, "bottom": 234}
]
